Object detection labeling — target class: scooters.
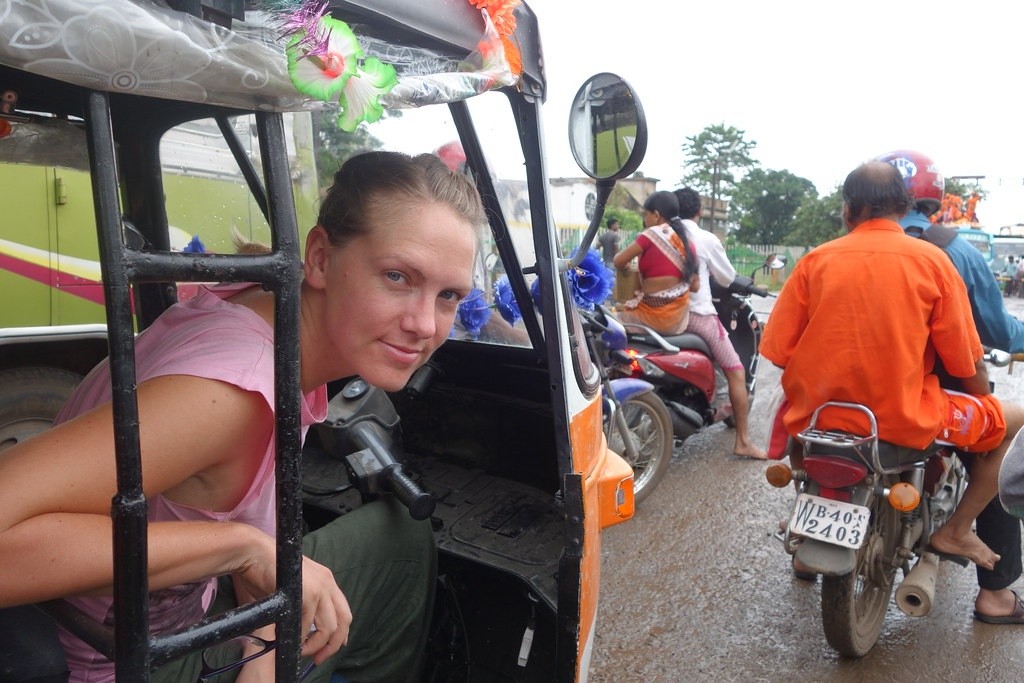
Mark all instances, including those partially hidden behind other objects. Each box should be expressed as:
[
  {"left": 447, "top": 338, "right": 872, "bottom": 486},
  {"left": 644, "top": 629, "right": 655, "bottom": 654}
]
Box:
[{"left": 591, "top": 251, "right": 787, "bottom": 450}]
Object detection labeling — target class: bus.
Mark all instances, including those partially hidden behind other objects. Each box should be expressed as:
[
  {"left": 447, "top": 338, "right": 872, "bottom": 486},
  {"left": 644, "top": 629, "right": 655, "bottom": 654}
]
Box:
[{"left": 950, "top": 221, "right": 1024, "bottom": 290}]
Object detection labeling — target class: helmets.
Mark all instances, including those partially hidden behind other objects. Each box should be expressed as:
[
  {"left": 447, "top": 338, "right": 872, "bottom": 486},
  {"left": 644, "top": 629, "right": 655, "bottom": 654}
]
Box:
[
  {"left": 433, "top": 139, "right": 478, "bottom": 190},
  {"left": 872, "top": 151, "right": 944, "bottom": 213}
]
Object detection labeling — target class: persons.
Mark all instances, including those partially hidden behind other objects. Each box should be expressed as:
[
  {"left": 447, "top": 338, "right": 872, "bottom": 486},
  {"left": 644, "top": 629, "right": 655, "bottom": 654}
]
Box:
[
  {"left": 0, "top": 151, "right": 481, "bottom": 683},
  {"left": 433, "top": 143, "right": 531, "bottom": 346},
  {"left": 886, "top": 153, "right": 1023, "bottom": 625},
  {"left": 929, "top": 187, "right": 981, "bottom": 223},
  {"left": 755, "top": 160, "right": 1023, "bottom": 569},
  {"left": 612, "top": 190, "right": 702, "bottom": 333},
  {"left": 596, "top": 218, "right": 621, "bottom": 270},
  {"left": 997, "top": 254, "right": 1023, "bottom": 298},
  {"left": 674, "top": 187, "right": 770, "bottom": 459}
]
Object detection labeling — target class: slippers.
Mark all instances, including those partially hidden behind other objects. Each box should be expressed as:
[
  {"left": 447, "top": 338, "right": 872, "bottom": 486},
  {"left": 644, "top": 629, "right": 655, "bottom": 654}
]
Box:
[{"left": 971, "top": 589, "right": 1022, "bottom": 626}]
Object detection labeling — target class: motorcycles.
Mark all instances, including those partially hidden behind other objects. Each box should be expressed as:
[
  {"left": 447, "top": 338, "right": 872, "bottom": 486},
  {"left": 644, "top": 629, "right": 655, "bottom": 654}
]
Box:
[
  {"left": 769, "top": 348, "right": 1023, "bottom": 661},
  {"left": 0, "top": 1, "right": 652, "bottom": 683}
]
